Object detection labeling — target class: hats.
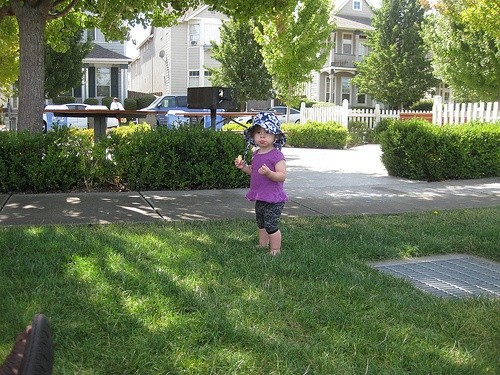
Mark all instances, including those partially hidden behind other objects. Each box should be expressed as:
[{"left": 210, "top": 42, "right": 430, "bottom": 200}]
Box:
[{"left": 244, "top": 112, "right": 287, "bottom": 166}]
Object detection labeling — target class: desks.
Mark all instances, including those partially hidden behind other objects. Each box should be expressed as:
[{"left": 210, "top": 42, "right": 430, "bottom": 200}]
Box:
[{"left": 52, "top": 109, "right": 147, "bottom": 168}]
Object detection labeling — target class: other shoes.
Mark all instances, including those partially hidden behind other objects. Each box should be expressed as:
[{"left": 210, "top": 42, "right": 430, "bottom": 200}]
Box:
[{"left": 0, "top": 314, "right": 52, "bottom": 375}]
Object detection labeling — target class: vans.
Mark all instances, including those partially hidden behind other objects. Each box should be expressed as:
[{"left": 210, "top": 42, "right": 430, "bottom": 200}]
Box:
[{"left": 137, "top": 95, "right": 226, "bottom": 130}]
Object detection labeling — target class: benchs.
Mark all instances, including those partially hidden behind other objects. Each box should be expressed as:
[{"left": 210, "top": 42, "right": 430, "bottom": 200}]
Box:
[{"left": 184, "top": 111, "right": 283, "bottom": 137}]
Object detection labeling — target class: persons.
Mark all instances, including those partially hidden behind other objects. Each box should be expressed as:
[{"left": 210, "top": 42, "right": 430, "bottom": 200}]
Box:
[
  {"left": 110, "top": 97, "right": 125, "bottom": 127},
  {"left": 233, "top": 112, "right": 288, "bottom": 255}
]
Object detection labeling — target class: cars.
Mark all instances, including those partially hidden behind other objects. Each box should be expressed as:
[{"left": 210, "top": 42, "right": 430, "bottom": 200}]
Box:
[
  {"left": 43, "top": 103, "right": 119, "bottom": 133},
  {"left": 230, "top": 106, "right": 301, "bottom": 124}
]
